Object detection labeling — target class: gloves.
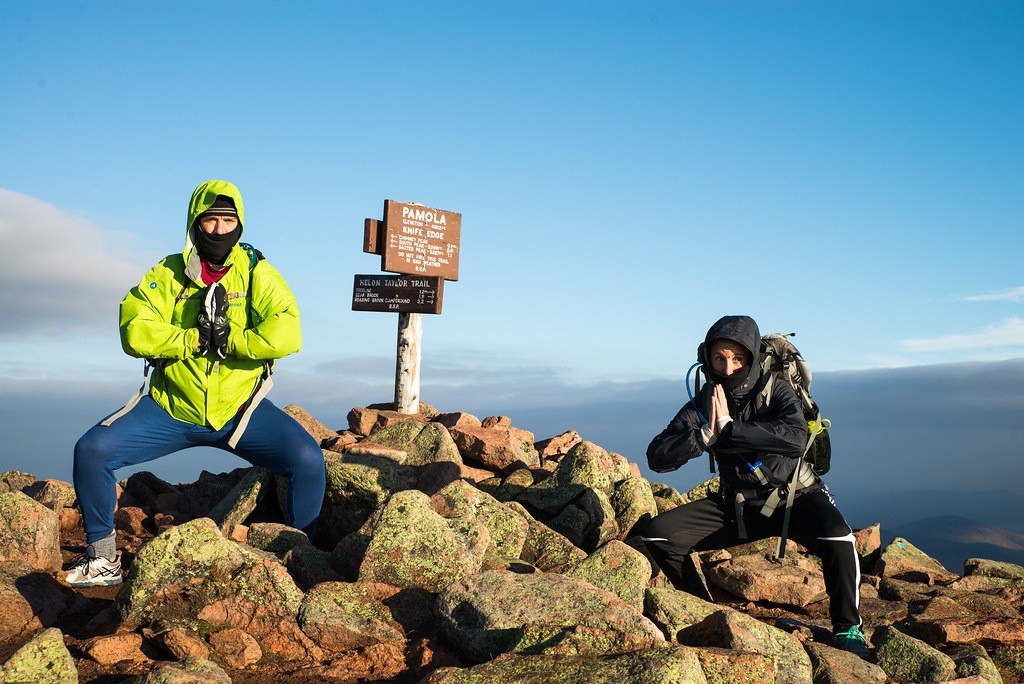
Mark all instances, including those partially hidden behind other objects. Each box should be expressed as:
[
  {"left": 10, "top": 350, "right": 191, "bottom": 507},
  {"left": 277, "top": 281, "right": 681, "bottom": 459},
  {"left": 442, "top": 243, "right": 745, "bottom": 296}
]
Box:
[
  {"left": 211, "top": 283, "right": 230, "bottom": 360},
  {"left": 196, "top": 282, "right": 219, "bottom": 357}
]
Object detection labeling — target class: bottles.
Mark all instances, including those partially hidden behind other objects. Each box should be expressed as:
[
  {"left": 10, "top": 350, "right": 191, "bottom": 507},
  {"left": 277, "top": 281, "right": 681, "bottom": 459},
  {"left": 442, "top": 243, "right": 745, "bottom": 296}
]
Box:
[{"left": 806, "top": 418, "right": 831, "bottom": 475}]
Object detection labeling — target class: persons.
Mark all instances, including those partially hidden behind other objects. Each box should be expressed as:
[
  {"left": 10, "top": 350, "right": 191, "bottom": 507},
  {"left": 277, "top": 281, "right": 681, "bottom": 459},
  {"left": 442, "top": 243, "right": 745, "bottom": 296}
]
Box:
[
  {"left": 639, "top": 314, "right": 872, "bottom": 659},
  {"left": 57, "top": 181, "right": 326, "bottom": 588}
]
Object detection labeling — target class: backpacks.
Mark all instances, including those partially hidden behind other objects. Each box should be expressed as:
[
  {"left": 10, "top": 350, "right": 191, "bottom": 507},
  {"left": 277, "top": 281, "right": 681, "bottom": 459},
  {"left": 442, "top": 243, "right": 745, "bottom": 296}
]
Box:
[{"left": 697, "top": 334, "right": 833, "bottom": 476}]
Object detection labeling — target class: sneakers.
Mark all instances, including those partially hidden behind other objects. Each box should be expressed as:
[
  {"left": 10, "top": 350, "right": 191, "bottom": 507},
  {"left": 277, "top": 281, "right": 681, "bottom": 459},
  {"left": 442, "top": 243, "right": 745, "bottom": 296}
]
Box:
[
  {"left": 833, "top": 625, "right": 871, "bottom": 660},
  {"left": 65, "top": 553, "right": 123, "bottom": 588}
]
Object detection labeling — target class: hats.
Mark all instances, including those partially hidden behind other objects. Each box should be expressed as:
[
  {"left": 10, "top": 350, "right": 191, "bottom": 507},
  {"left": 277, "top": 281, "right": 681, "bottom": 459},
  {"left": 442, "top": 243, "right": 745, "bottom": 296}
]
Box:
[{"left": 192, "top": 195, "right": 239, "bottom": 225}]
